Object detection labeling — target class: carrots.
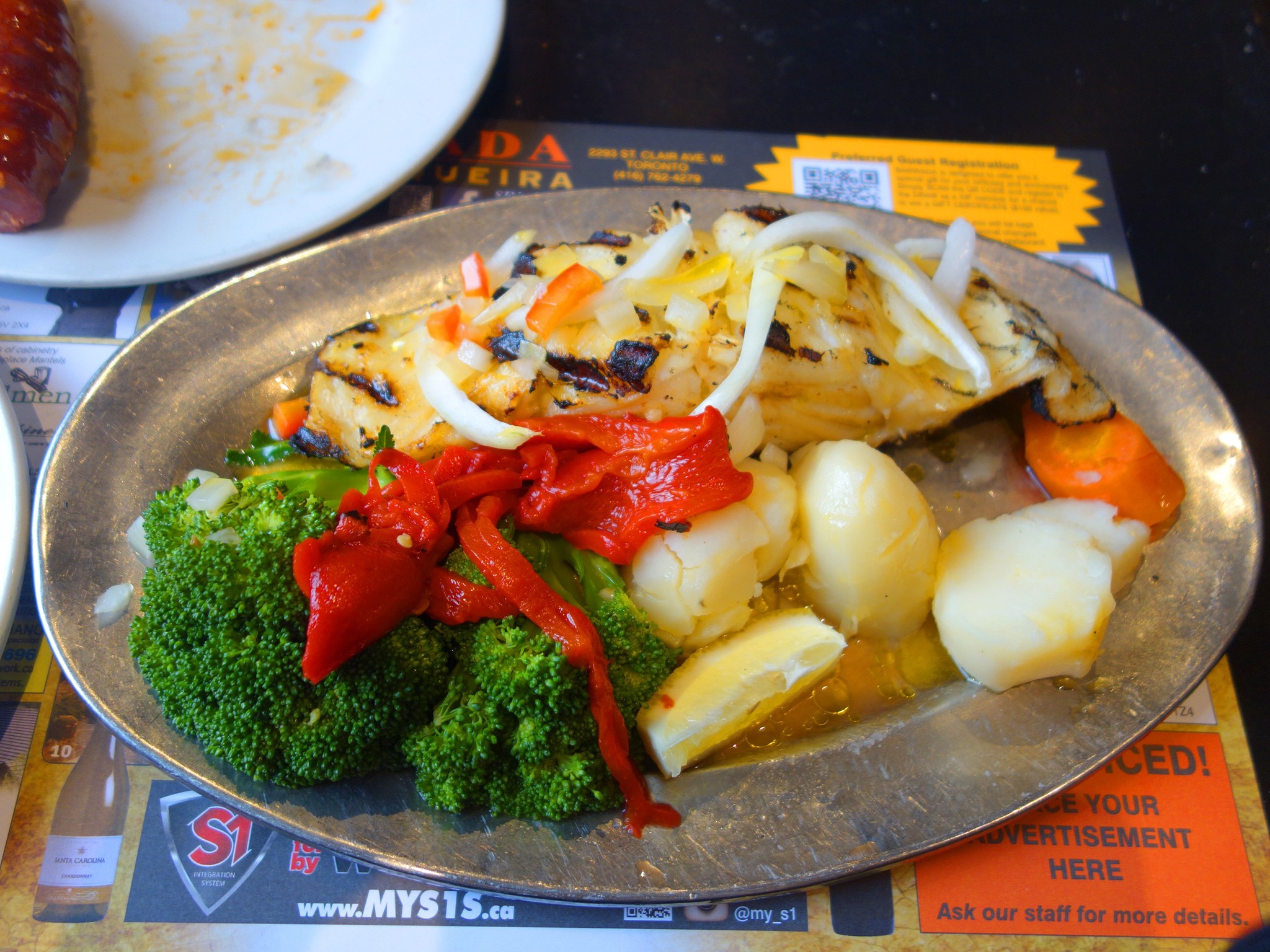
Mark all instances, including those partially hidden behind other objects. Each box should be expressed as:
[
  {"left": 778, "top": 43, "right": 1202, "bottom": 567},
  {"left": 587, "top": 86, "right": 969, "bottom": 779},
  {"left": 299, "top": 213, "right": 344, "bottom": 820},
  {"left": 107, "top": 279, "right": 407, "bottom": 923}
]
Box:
[{"left": 1017, "top": 387, "right": 1188, "bottom": 533}]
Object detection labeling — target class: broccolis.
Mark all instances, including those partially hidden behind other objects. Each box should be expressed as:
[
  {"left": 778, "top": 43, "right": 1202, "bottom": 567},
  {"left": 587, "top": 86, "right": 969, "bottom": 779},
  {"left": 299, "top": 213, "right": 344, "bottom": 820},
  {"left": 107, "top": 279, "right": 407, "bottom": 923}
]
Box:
[{"left": 136, "top": 448, "right": 684, "bottom": 834}]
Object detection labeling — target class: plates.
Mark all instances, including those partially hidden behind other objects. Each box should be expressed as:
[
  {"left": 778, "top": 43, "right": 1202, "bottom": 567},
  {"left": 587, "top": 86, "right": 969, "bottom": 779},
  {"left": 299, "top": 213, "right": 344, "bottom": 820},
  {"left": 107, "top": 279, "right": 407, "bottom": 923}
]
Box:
[
  {"left": 0, "top": 0, "right": 507, "bottom": 291},
  {"left": 0, "top": 377, "right": 27, "bottom": 653},
  {"left": 31, "top": 188, "right": 1260, "bottom": 903}
]
